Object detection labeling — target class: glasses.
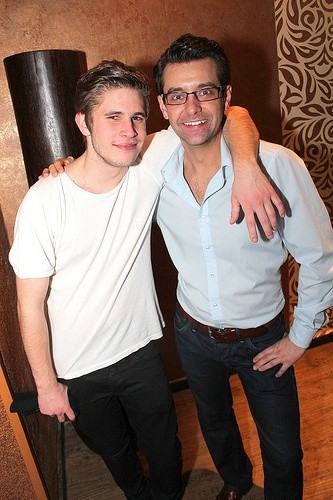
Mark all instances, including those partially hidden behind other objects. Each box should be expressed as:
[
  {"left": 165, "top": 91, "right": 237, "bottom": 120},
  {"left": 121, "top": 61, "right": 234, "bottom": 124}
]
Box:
[{"left": 162, "top": 85, "right": 226, "bottom": 105}]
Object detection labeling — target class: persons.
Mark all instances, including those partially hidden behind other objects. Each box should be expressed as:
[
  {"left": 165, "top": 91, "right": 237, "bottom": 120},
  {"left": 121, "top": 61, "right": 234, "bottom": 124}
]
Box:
[
  {"left": 39, "top": 34, "right": 333, "bottom": 500},
  {"left": 7, "top": 59, "right": 287, "bottom": 499}
]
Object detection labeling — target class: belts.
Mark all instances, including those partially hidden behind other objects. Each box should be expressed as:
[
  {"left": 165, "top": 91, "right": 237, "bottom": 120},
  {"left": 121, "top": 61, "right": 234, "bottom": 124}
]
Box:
[{"left": 176, "top": 301, "right": 283, "bottom": 342}]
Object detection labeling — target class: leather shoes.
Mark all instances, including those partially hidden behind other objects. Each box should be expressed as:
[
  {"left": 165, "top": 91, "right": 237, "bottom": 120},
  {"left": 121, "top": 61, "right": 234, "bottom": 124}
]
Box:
[{"left": 216, "top": 485, "right": 253, "bottom": 500}]
